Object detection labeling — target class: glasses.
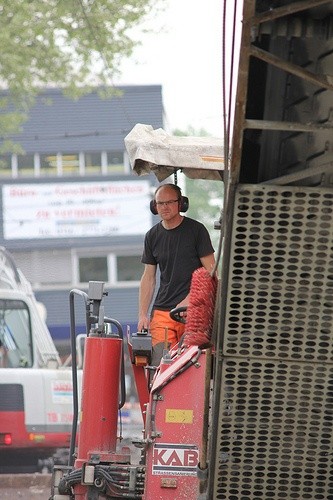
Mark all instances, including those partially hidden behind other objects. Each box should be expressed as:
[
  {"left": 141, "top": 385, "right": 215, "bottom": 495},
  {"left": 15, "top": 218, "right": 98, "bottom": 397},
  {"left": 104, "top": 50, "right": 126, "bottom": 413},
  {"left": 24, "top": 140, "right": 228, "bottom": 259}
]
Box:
[{"left": 154, "top": 200, "right": 179, "bottom": 205}]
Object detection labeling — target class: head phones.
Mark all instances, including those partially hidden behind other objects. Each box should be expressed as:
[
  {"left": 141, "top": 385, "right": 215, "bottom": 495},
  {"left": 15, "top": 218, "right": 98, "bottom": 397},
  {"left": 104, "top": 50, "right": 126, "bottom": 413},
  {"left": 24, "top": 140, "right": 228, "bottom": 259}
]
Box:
[{"left": 149, "top": 196, "right": 189, "bottom": 215}]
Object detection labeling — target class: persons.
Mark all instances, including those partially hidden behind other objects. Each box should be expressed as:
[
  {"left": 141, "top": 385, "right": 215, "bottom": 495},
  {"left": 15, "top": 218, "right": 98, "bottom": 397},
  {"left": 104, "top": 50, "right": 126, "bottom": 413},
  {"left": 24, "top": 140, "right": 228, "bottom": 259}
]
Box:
[{"left": 136, "top": 184, "right": 218, "bottom": 351}]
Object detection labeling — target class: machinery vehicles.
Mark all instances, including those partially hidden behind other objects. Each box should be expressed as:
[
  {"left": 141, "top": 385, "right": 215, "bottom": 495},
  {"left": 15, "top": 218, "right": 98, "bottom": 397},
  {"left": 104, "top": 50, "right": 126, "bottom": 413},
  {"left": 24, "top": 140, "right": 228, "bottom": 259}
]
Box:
[{"left": 0, "top": 246, "right": 145, "bottom": 474}]
